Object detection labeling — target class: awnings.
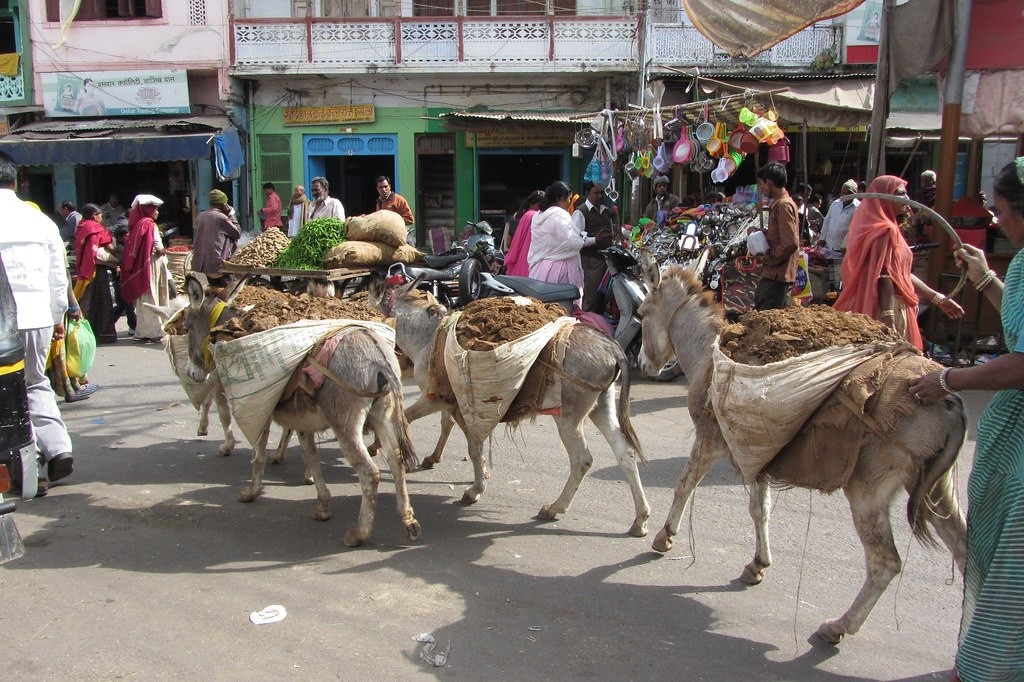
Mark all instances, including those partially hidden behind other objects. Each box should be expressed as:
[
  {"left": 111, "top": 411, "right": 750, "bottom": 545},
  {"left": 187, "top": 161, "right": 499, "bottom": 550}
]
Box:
[
  {"left": 0, "top": 115, "right": 242, "bottom": 180},
  {"left": 438, "top": 73, "right": 879, "bottom": 129}
]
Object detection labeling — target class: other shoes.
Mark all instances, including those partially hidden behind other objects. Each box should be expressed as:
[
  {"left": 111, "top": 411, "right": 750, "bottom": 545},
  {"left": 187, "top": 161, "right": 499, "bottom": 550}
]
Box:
[
  {"left": 36, "top": 478, "right": 48, "bottom": 497},
  {"left": 48, "top": 452, "right": 73, "bottom": 482},
  {"left": 65, "top": 383, "right": 100, "bottom": 403}
]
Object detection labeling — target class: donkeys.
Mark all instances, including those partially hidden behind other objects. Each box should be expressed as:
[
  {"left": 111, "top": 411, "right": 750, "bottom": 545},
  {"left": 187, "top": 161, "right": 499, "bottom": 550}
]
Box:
[
  {"left": 182, "top": 271, "right": 421, "bottom": 545},
  {"left": 638, "top": 259, "right": 968, "bottom": 645},
  {"left": 369, "top": 269, "right": 651, "bottom": 538}
]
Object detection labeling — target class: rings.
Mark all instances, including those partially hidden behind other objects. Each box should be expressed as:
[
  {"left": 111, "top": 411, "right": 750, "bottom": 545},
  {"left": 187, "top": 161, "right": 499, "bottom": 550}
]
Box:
[{"left": 915, "top": 393, "right": 921, "bottom": 400}]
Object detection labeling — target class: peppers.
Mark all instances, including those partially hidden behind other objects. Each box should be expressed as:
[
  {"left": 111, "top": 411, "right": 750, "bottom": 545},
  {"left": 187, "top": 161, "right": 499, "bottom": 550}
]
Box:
[{"left": 168, "top": 244, "right": 193, "bottom": 252}]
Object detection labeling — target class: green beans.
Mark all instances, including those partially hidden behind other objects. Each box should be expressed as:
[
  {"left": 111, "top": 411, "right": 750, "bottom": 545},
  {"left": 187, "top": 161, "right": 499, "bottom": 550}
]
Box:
[{"left": 272, "top": 217, "right": 346, "bottom": 271}]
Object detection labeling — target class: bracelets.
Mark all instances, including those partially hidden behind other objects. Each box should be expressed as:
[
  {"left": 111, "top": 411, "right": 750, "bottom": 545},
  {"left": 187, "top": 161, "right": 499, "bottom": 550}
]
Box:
[
  {"left": 940, "top": 367, "right": 961, "bottom": 394},
  {"left": 973, "top": 270, "right": 997, "bottom": 292},
  {"left": 931, "top": 292, "right": 945, "bottom": 305},
  {"left": 261, "top": 209, "right": 263, "bottom": 213},
  {"left": 68, "top": 305, "right": 78, "bottom": 311},
  {"left": 881, "top": 310, "right": 895, "bottom": 317}
]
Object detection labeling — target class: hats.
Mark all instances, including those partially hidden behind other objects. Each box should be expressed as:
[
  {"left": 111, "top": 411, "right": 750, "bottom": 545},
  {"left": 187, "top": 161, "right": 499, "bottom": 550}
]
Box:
[
  {"left": 467, "top": 220, "right": 493, "bottom": 235},
  {"left": 653, "top": 175, "right": 670, "bottom": 190},
  {"left": 209, "top": 188, "right": 228, "bottom": 205},
  {"left": 921, "top": 170, "right": 936, "bottom": 189},
  {"left": 843, "top": 179, "right": 858, "bottom": 194}
]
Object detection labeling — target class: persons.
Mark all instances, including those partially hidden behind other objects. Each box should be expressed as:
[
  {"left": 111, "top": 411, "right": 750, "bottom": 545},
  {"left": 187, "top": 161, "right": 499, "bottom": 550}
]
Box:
[
  {"left": 75, "top": 78, "right": 105, "bottom": 114},
  {"left": 309, "top": 177, "right": 346, "bottom": 222},
  {"left": 791, "top": 169, "right": 994, "bottom": 255},
  {"left": 64, "top": 86, "right": 73, "bottom": 99},
  {"left": 258, "top": 182, "right": 283, "bottom": 230},
  {"left": 643, "top": 175, "right": 681, "bottom": 221},
  {"left": 376, "top": 176, "right": 414, "bottom": 225},
  {"left": 527, "top": 181, "right": 587, "bottom": 310},
  {"left": 287, "top": 185, "right": 311, "bottom": 238},
  {"left": 907, "top": 155, "right": 1024, "bottom": 682},
  {"left": 570, "top": 180, "right": 612, "bottom": 317},
  {"left": 833, "top": 176, "right": 965, "bottom": 350},
  {"left": 746, "top": 161, "right": 799, "bottom": 310},
  {"left": 0, "top": 152, "right": 73, "bottom": 495},
  {"left": 24, "top": 195, "right": 176, "bottom": 404},
  {"left": 193, "top": 189, "right": 241, "bottom": 288},
  {"left": 506, "top": 190, "right": 545, "bottom": 278}
]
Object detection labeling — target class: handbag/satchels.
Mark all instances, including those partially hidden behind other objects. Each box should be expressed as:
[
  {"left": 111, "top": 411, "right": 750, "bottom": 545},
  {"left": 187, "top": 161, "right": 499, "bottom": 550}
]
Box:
[{"left": 65, "top": 318, "right": 96, "bottom": 378}]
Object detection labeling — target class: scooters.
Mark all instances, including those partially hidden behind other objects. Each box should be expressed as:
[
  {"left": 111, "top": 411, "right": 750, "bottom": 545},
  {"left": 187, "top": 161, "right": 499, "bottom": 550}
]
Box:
[{"left": 384, "top": 219, "right": 684, "bottom": 383}]
